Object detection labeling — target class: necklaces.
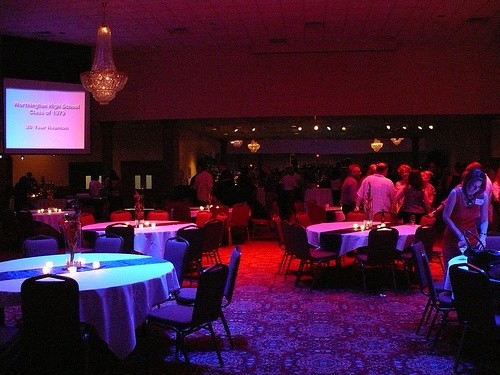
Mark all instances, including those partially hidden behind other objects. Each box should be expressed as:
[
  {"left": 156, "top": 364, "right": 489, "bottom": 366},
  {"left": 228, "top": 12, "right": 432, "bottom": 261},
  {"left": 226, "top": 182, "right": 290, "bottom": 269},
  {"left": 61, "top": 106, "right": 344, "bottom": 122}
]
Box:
[{"left": 461, "top": 188, "right": 477, "bottom": 206}]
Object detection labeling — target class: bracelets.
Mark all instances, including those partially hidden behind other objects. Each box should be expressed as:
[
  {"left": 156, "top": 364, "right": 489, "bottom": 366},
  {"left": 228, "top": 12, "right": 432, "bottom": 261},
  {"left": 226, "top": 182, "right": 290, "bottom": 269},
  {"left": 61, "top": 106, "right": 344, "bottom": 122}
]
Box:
[{"left": 479, "top": 233, "right": 487, "bottom": 236}]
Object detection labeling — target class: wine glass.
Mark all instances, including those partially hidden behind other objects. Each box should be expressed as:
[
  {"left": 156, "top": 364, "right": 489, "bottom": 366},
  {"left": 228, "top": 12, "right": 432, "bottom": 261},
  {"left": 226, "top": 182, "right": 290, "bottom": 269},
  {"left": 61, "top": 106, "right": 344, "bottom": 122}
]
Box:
[{"left": 457, "top": 241, "right": 468, "bottom": 260}]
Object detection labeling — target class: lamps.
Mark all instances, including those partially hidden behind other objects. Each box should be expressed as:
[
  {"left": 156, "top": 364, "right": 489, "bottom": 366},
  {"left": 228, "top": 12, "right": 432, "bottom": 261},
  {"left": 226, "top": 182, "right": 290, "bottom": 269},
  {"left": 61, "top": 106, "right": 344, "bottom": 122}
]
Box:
[
  {"left": 229, "top": 124, "right": 262, "bottom": 153},
  {"left": 80, "top": 2, "right": 129, "bottom": 105},
  {"left": 370, "top": 138, "right": 384, "bottom": 153},
  {"left": 389, "top": 138, "right": 405, "bottom": 145}
]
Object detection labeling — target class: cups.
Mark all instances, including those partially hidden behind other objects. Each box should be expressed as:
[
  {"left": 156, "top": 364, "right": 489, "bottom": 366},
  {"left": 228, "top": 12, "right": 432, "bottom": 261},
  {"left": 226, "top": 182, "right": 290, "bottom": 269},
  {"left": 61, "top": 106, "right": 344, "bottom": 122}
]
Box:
[
  {"left": 199, "top": 205, "right": 219, "bottom": 211},
  {"left": 126, "top": 220, "right": 156, "bottom": 230},
  {"left": 352, "top": 221, "right": 385, "bottom": 231},
  {"left": 38, "top": 207, "right": 62, "bottom": 214},
  {"left": 43, "top": 257, "right": 100, "bottom": 276}
]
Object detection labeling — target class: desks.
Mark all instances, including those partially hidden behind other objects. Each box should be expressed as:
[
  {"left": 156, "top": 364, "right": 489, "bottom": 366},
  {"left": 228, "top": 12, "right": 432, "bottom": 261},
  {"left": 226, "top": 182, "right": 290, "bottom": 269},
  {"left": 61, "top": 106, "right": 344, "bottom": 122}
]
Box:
[
  {"left": 32, "top": 198, "right": 69, "bottom": 208},
  {"left": 0, "top": 252, "right": 181, "bottom": 361},
  {"left": 29, "top": 208, "right": 66, "bottom": 233},
  {"left": 305, "top": 188, "right": 333, "bottom": 209},
  {"left": 305, "top": 220, "right": 420, "bottom": 289},
  {"left": 80, "top": 220, "right": 198, "bottom": 259},
  {"left": 443, "top": 255, "right": 466, "bottom": 298}
]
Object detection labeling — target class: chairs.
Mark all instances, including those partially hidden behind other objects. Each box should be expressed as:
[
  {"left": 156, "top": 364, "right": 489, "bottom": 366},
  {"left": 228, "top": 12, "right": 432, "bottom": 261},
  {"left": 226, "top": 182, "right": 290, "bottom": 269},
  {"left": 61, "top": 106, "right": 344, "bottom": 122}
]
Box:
[
  {"left": 17, "top": 274, "right": 98, "bottom": 375},
  {"left": 0, "top": 298, "right": 25, "bottom": 375},
  {"left": 147, "top": 200, "right": 500, "bottom": 375},
  {"left": 0, "top": 191, "right": 135, "bottom": 257}
]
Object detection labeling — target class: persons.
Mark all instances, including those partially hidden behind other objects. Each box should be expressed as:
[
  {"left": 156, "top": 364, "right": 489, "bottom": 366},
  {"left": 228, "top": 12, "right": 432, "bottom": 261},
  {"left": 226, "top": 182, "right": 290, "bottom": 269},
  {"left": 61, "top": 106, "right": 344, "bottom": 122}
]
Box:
[
  {"left": 13, "top": 158, "right": 500, "bottom": 307},
  {"left": 441, "top": 167, "right": 490, "bottom": 293}
]
